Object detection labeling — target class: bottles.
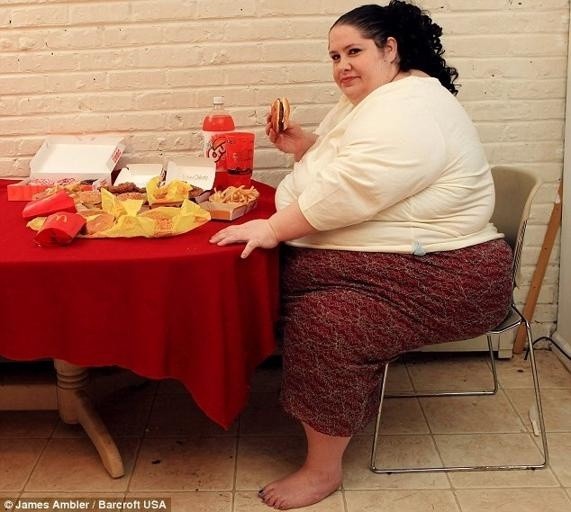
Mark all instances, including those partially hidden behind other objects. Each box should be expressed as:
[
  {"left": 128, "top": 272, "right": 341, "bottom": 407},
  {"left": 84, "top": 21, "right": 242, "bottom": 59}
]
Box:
[{"left": 203, "top": 96, "right": 236, "bottom": 190}]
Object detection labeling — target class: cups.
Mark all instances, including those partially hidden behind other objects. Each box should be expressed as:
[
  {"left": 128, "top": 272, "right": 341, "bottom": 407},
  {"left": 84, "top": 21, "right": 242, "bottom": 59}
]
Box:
[{"left": 225, "top": 132, "right": 254, "bottom": 189}]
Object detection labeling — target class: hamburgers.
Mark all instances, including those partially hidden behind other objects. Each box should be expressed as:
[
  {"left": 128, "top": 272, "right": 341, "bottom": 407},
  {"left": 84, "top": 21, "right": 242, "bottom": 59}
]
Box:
[{"left": 271, "top": 96, "right": 290, "bottom": 133}]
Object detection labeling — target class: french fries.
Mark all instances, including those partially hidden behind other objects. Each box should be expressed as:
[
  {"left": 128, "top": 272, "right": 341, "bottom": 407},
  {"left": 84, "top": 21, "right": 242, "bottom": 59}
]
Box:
[{"left": 210, "top": 186, "right": 260, "bottom": 206}]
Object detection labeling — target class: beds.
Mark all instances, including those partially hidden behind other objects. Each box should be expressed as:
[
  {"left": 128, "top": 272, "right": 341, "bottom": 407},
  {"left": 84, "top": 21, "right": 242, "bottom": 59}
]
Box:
[{"left": 1, "top": 169, "right": 280, "bottom": 480}]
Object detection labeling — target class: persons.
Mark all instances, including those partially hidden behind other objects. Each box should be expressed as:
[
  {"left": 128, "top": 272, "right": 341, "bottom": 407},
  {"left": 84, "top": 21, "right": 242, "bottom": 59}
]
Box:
[{"left": 207, "top": 1, "right": 517, "bottom": 511}]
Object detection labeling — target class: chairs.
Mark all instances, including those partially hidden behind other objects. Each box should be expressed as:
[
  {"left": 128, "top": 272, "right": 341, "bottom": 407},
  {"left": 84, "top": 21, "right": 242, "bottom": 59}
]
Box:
[{"left": 368, "top": 165, "right": 550, "bottom": 473}]
